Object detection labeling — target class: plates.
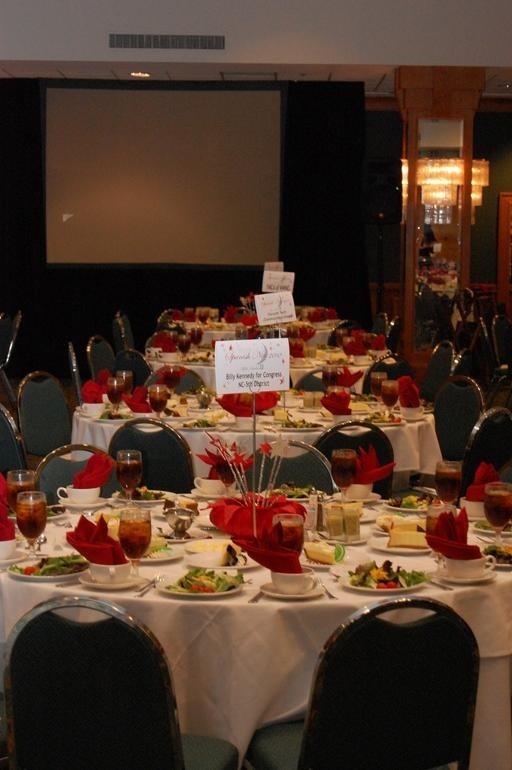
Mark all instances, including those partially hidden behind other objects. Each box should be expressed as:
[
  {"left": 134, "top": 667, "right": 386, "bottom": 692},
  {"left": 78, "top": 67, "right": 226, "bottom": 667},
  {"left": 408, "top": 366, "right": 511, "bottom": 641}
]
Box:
[
  {"left": 164, "top": 527, "right": 212, "bottom": 543},
  {"left": 75, "top": 406, "right": 82, "bottom": 412},
  {"left": 467, "top": 516, "right": 488, "bottom": 522},
  {"left": 298, "top": 408, "right": 322, "bottom": 414},
  {"left": 142, "top": 356, "right": 159, "bottom": 361},
  {"left": 181, "top": 391, "right": 197, "bottom": 398},
  {"left": 156, "top": 573, "right": 243, "bottom": 598},
  {"left": 6, "top": 560, "right": 89, "bottom": 582},
  {"left": 161, "top": 416, "right": 194, "bottom": 421},
  {"left": 277, "top": 397, "right": 302, "bottom": 407},
  {"left": 182, "top": 360, "right": 208, "bottom": 366},
  {"left": 77, "top": 573, "right": 144, "bottom": 590},
  {"left": 184, "top": 553, "right": 260, "bottom": 569},
  {"left": 259, "top": 582, "right": 324, "bottom": 601},
  {"left": 383, "top": 500, "right": 427, "bottom": 513},
  {"left": 353, "top": 410, "right": 371, "bottom": 416},
  {"left": 484, "top": 560, "right": 512, "bottom": 569},
  {"left": 366, "top": 536, "right": 432, "bottom": 555},
  {"left": 328, "top": 565, "right": 355, "bottom": 577},
  {"left": 58, "top": 497, "right": 108, "bottom": 509},
  {"left": 8, "top": 509, "right": 68, "bottom": 520},
  {"left": 186, "top": 406, "right": 215, "bottom": 413},
  {"left": 184, "top": 538, "right": 245, "bottom": 554},
  {"left": 332, "top": 492, "right": 382, "bottom": 503},
  {"left": 279, "top": 422, "right": 324, "bottom": 432},
  {"left": 95, "top": 417, "right": 136, "bottom": 424},
  {"left": 299, "top": 559, "right": 334, "bottom": 569},
  {"left": 327, "top": 538, "right": 368, "bottom": 546},
  {"left": 338, "top": 576, "right": 427, "bottom": 592},
  {"left": 352, "top": 361, "right": 370, "bottom": 366},
  {"left": 112, "top": 489, "right": 179, "bottom": 504},
  {"left": 369, "top": 419, "right": 404, "bottom": 426},
  {"left": 79, "top": 412, "right": 97, "bottom": 418},
  {"left": 174, "top": 424, "right": 222, "bottom": 432},
  {"left": 391, "top": 408, "right": 434, "bottom": 413},
  {"left": 360, "top": 401, "right": 378, "bottom": 406},
  {"left": 159, "top": 359, "right": 181, "bottom": 364},
  {"left": 471, "top": 524, "right": 512, "bottom": 536},
  {"left": 140, "top": 544, "right": 185, "bottom": 563},
  {"left": 289, "top": 363, "right": 314, "bottom": 369},
  {"left": 359, "top": 507, "right": 379, "bottom": 522},
  {"left": 370, "top": 527, "right": 390, "bottom": 536},
  {"left": 261, "top": 488, "right": 326, "bottom": 501},
  {"left": 0, "top": 549, "right": 26, "bottom": 569},
  {"left": 436, "top": 570, "right": 498, "bottom": 583},
  {"left": 400, "top": 414, "right": 428, "bottom": 421},
  {"left": 190, "top": 488, "right": 227, "bottom": 498},
  {"left": 231, "top": 426, "right": 261, "bottom": 432}
]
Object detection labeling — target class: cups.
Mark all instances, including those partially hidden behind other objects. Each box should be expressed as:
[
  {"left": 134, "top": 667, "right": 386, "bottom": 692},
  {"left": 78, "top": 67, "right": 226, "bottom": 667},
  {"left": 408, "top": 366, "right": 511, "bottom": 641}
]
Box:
[
  {"left": 400, "top": 406, "right": 426, "bottom": 417},
  {"left": 290, "top": 358, "right": 305, "bottom": 366},
  {"left": 422, "top": 203, "right": 454, "bottom": 225},
  {"left": 80, "top": 403, "right": 107, "bottom": 415},
  {"left": 100, "top": 394, "right": 110, "bottom": 404},
  {"left": 346, "top": 482, "right": 374, "bottom": 499},
  {"left": 157, "top": 352, "right": 178, "bottom": 361},
  {"left": 88, "top": 560, "right": 132, "bottom": 583},
  {"left": 353, "top": 355, "right": 371, "bottom": 363},
  {"left": 441, "top": 555, "right": 498, "bottom": 578},
  {"left": 145, "top": 347, "right": 163, "bottom": 357},
  {"left": 133, "top": 412, "right": 152, "bottom": 420},
  {"left": 342, "top": 387, "right": 350, "bottom": 394},
  {"left": 235, "top": 416, "right": 256, "bottom": 427},
  {"left": 184, "top": 321, "right": 196, "bottom": 329},
  {"left": 193, "top": 475, "right": 236, "bottom": 494},
  {"left": 208, "top": 308, "right": 219, "bottom": 324},
  {"left": 0, "top": 538, "right": 17, "bottom": 560},
  {"left": 369, "top": 350, "right": 392, "bottom": 357},
  {"left": 331, "top": 414, "right": 351, "bottom": 424},
  {"left": 270, "top": 566, "right": 318, "bottom": 594},
  {"left": 459, "top": 497, "right": 485, "bottom": 517},
  {"left": 55, "top": 484, "right": 101, "bottom": 504}
]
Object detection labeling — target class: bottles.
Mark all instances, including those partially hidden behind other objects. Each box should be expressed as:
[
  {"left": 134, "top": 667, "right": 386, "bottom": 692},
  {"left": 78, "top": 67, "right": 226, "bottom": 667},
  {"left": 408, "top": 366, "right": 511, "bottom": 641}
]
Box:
[
  {"left": 303, "top": 492, "right": 317, "bottom": 546},
  {"left": 314, "top": 391, "right": 324, "bottom": 409},
  {"left": 302, "top": 391, "right": 313, "bottom": 409},
  {"left": 320, "top": 504, "right": 345, "bottom": 541},
  {"left": 341, "top": 502, "right": 362, "bottom": 540}
]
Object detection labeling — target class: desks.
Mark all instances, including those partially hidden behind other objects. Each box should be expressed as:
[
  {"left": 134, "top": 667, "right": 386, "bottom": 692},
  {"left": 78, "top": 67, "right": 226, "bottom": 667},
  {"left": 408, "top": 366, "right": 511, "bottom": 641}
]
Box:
[{"left": 0, "top": 491, "right": 512, "bottom": 770}]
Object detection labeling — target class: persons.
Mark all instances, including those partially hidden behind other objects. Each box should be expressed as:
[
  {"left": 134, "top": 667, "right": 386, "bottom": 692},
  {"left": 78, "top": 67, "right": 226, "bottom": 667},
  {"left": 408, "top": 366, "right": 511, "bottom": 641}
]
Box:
[{"left": 405, "top": 222, "right": 438, "bottom": 271}]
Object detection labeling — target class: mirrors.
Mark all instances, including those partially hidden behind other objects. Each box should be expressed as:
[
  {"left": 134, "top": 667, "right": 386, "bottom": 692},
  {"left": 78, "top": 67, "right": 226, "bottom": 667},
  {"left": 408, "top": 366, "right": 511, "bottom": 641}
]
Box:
[{"left": 416, "top": 117, "right": 464, "bottom": 345}]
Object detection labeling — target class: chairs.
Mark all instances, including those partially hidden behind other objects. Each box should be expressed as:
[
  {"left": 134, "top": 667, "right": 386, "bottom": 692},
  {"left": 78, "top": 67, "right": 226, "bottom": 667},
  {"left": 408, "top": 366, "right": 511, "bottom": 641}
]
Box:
[
  {"left": 0, "top": 593, "right": 239, "bottom": 770},
  {"left": 0, "top": 288, "right": 512, "bottom": 506},
  {"left": 245, "top": 597, "right": 479, "bottom": 770}
]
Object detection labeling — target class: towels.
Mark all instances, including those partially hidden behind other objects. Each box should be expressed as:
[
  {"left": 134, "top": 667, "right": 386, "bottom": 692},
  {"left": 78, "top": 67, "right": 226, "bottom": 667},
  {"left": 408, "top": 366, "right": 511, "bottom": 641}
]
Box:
[
  {"left": 229, "top": 524, "right": 305, "bottom": 573},
  {"left": 0, "top": 472, "right": 16, "bottom": 542},
  {"left": 64, "top": 515, "right": 127, "bottom": 566},
  {"left": 424, "top": 507, "right": 483, "bottom": 560}
]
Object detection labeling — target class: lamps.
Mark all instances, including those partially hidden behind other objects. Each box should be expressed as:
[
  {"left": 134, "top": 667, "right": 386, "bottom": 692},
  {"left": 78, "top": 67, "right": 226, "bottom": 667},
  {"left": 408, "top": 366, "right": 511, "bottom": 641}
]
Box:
[
  {"left": 416, "top": 158, "right": 464, "bottom": 224},
  {"left": 417, "top": 158, "right": 464, "bottom": 224},
  {"left": 471, "top": 158, "right": 489, "bottom": 225}
]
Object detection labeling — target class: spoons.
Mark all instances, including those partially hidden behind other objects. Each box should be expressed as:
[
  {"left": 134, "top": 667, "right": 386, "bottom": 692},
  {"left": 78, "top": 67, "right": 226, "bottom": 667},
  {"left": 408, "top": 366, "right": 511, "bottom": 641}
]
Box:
[
  {"left": 36, "top": 534, "right": 46, "bottom": 551},
  {"left": 133, "top": 576, "right": 160, "bottom": 593}
]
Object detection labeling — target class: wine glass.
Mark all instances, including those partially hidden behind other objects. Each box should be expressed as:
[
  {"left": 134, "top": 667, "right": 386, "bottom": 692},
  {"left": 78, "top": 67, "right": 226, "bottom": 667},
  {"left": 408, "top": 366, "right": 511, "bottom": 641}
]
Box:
[
  {"left": 177, "top": 334, "right": 191, "bottom": 357},
  {"left": 116, "top": 449, "right": 143, "bottom": 509},
  {"left": 16, "top": 491, "right": 48, "bottom": 561},
  {"left": 322, "top": 366, "right": 337, "bottom": 393},
  {"left": 326, "top": 385, "right": 343, "bottom": 398},
  {"left": 362, "top": 333, "right": 373, "bottom": 350},
  {"left": 434, "top": 459, "right": 464, "bottom": 515},
  {"left": 330, "top": 448, "right": 357, "bottom": 503},
  {"left": 190, "top": 326, "right": 203, "bottom": 348},
  {"left": 116, "top": 370, "right": 134, "bottom": 396},
  {"left": 148, "top": 384, "right": 168, "bottom": 421},
  {"left": 6, "top": 470, "right": 36, "bottom": 514},
  {"left": 161, "top": 366, "right": 177, "bottom": 394},
  {"left": 107, "top": 377, "right": 125, "bottom": 415},
  {"left": 380, "top": 380, "right": 399, "bottom": 413},
  {"left": 117, "top": 509, "right": 152, "bottom": 577},
  {"left": 165, "top": 331, "right": 177, "bottom": 344},
  {"left": 481, "top": 482, "right": 512, "bottom": 548},
  {"left": 342, "top": 336, "right": 355, "bottom": 359},
  {"left": 214, "top": 444, "right": 241, "bottom": 496},
  {"left": 370, "top": 371, "right": 388, "bottom": 399},
  {"left": 335, "top": 328, "right": 348, "bottom": 348},
  {"left": 270, "top": 513, "right": 305, "bottom": 560},
  {"left": 197, "top": 308, "right": 209, "bottom": 324}
]
